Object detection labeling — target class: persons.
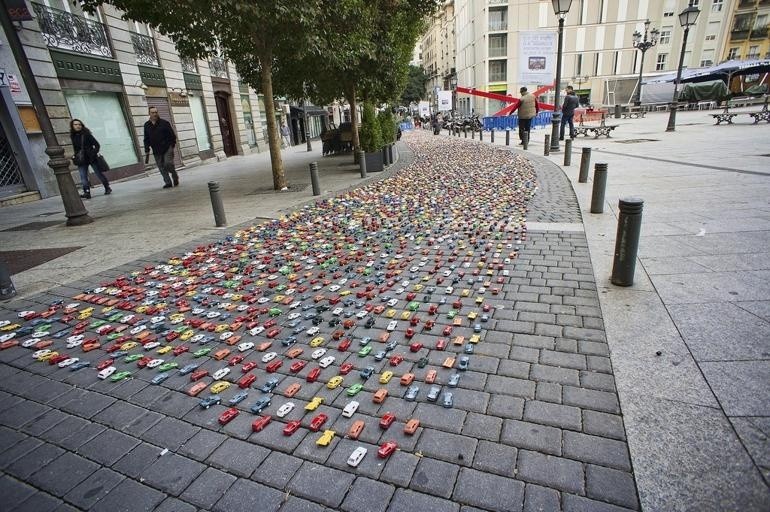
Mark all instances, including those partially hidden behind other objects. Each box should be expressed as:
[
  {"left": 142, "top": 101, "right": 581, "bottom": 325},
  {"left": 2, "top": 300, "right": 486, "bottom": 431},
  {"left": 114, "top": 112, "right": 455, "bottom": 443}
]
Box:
[
  {"left": 558, "top": 86, "right": 580, "bottom": 141},
  {"left": 143, "top": 106, "right": 181, "bottom": 190},
  {"left": 505, "top": 87, "right": 540, "bottom": 147},
  {"left": 280, "top": 121, "right": 292, "bottom": 149},
  {"left": 398, "top": 111, "right": 445, "bottom": 135},
  {"left": 70, "top": 119, "right": 113, "bottom": 199}
]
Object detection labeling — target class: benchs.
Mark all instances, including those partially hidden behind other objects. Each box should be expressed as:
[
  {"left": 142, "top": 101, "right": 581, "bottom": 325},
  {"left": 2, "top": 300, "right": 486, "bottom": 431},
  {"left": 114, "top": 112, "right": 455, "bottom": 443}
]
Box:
[
  {"left": 573, "top": 112, "right": 618, "bottom": 140},
  {"left": 622, "top": 96, "right": 769, "bottom": 125}
]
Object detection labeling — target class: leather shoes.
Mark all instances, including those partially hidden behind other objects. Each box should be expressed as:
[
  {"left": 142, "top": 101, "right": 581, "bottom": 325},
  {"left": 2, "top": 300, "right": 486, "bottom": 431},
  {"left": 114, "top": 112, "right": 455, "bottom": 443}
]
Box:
[{"left": 163, "top": 177, "right": 178, "bottom": 188}]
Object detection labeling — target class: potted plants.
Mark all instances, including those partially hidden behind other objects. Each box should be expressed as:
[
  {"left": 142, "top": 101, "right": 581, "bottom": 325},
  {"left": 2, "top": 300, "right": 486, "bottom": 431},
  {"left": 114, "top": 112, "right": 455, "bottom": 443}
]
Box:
[
  {"left": 358, "top": 103, "right": 383, "bottom": 171},
  {"left": 378, "top": 106, "right": 398, "bottom": 166}
]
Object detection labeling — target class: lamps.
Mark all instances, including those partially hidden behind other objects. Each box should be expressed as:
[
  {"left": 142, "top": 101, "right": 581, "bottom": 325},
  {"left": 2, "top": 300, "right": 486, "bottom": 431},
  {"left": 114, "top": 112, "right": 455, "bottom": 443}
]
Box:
[
  {"left": 134, "top": 79, "right": 149, "bottom": 90},
  {"left": 173, "top": 87, "right": 193, "bottom": 97}
]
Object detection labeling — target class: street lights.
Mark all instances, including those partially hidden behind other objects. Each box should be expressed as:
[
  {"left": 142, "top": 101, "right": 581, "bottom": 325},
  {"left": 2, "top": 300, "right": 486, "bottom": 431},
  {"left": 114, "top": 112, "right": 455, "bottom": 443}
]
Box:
[
  {"left": 665, "top": 2, "right": 701, "bottom": 132},
  {"left": 549, "top": 0, "right": 574, "bottom": 151},
  {"left": 633, "top": 18, "right": 661, "bottom": 112},
  {"left": 448, "top": 74, "right": 458, "bottom": 121}
]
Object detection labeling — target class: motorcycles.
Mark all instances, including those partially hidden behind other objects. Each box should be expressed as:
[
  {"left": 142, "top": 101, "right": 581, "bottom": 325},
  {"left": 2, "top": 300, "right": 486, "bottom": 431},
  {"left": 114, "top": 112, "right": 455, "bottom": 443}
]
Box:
[{"left": 424, "top": 107, "right": 484, "bottom": 132}]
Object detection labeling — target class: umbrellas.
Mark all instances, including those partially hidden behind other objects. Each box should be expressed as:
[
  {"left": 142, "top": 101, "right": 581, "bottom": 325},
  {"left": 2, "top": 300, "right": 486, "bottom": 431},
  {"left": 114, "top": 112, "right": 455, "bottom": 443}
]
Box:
[{"left": 636, "top": 57, "right": 770, "bottom": 102}]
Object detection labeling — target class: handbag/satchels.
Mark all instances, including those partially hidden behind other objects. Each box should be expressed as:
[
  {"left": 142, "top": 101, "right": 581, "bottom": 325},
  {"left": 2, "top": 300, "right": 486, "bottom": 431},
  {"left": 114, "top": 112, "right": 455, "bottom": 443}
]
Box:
[
  {"left": 96, "top": 155, "right": 109, "bottom": 172},
  {"left": 71, "top": 150, "right": 85, "bottom": 165}
]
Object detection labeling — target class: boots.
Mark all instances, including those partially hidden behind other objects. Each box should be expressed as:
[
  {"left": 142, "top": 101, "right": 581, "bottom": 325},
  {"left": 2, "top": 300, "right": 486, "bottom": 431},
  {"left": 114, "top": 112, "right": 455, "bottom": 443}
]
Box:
[
  {"left": 103, "top": 181, "right": 112, "bottom": 194},
  {"left": 81, "top": 187, "right": 91, "bottom": 199}
]
